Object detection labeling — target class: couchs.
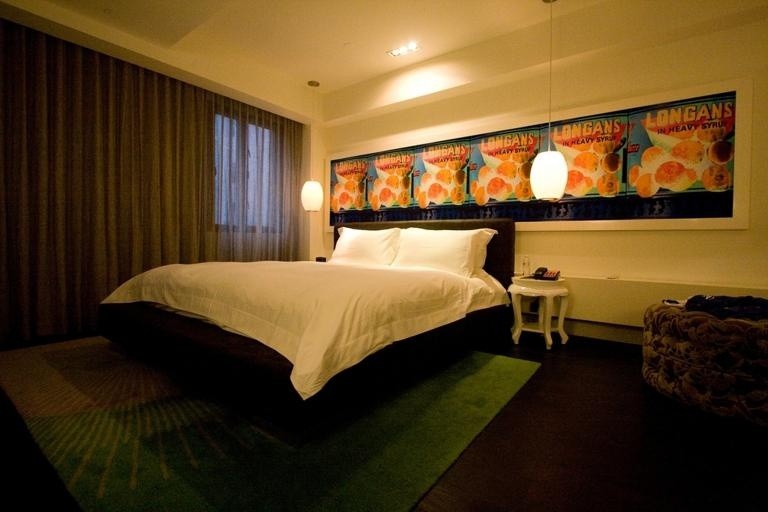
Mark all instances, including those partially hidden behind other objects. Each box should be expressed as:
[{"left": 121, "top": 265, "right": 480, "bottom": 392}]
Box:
[{"left": 642, "top": 301, "right": 768, "bottom": 417}]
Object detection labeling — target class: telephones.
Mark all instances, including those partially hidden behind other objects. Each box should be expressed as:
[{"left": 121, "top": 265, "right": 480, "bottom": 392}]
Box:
[{"left": 533, "top": 268, "right": 560, "bottom": 280}]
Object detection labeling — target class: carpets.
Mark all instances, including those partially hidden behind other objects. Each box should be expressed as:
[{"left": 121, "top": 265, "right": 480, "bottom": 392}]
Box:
[{"left": 0, "top": 333, "right": 540, "bottom": 511}]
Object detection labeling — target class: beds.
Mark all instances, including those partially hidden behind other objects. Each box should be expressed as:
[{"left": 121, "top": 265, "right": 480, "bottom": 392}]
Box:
[{"left": 100, "top": 217, "right": 515, "bottom": 460}]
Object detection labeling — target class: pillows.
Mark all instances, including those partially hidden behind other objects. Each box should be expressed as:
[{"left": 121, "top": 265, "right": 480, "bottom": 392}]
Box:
[{"left": 327, "top": 225, "right": 498, "bottom": 279}]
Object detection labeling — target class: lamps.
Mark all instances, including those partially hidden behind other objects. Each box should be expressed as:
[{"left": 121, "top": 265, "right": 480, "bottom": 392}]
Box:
[
  {"left": 300, "top": 88, "right": 324, "bottom": 213},
  {"left": 529, "top": 0, "right": 570, "bottom": 203}
]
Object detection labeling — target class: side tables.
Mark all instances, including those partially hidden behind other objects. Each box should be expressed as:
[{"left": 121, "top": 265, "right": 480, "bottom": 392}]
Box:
[{"left": 504, "top": 273, "right": 570, "bottom": 350}]
